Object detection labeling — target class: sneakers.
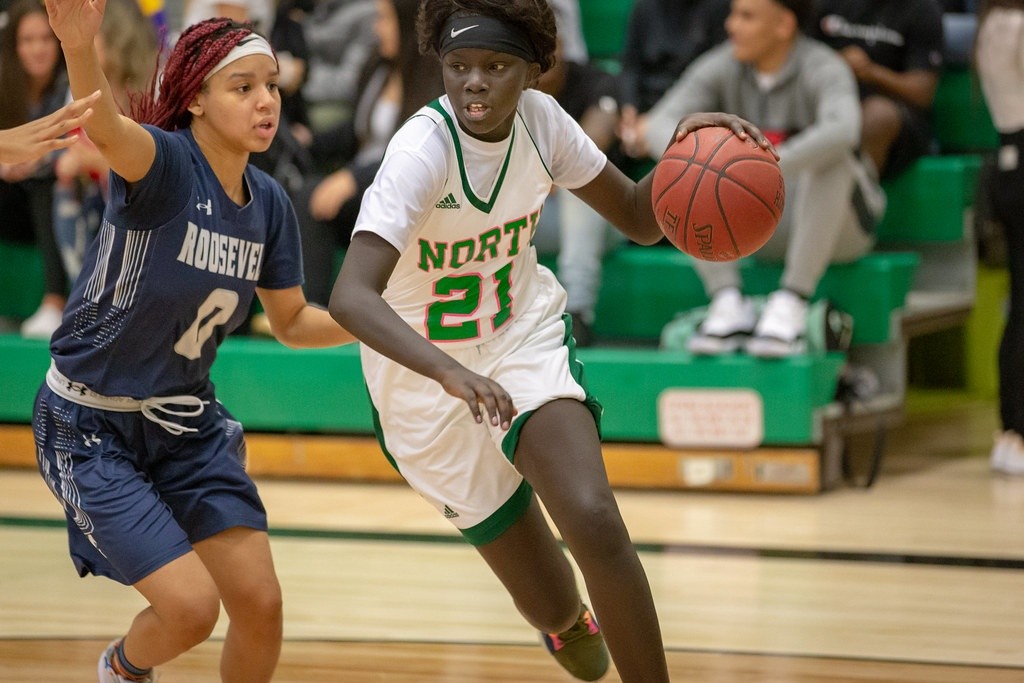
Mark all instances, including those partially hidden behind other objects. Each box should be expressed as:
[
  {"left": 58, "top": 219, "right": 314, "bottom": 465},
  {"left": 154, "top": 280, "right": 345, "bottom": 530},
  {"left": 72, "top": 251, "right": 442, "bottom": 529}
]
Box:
[
  {"left": 749, "top": 311, "right": 810, "bottom": 357},
  {"left": 687, "top": 305, "right": 756, "bottom": 355},
  {"left": 97, "top": 637, "right": 154, "bottom": 683},
  {"left": 540, "top": 602, "right": 610, "bottom": 682}
]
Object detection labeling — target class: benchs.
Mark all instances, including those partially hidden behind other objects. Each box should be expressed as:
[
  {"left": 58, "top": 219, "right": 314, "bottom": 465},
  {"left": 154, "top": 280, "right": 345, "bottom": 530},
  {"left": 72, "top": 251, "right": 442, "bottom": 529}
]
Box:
[{"left": 0, "top": 0, "right": 1000, "bottom": 494}]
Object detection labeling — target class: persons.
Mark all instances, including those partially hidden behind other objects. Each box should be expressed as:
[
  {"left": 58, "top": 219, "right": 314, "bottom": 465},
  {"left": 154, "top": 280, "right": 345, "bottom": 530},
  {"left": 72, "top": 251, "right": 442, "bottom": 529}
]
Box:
[
  {"left": 530, "top": 36, "right": 621, "bottom": 347},
  {"left": 807, "top": 0, "right": 947, "bottom": 182},
  {"left": 0, "top": 1, "right": 74, "bottom": 343},
  {"left": 33, "top": 0, "right": 359, "bottom": 683},
  {"left": 292, "top": 2, "right": 421, "bottom": 305},
  {"left": 271, "top": 1, "right": 398, "bottom": 103},
  {"left": 972, "top": 0, "right": 1024, "bottom": 474},
  {"left": 329, "top": 2, "right": 781, "bottom": 683},
  {"left": 327, "top": 0, "right": 781, "bottom": 682},
  {"left": 636, "top": 0, "right": 886, "bottom": 356}
]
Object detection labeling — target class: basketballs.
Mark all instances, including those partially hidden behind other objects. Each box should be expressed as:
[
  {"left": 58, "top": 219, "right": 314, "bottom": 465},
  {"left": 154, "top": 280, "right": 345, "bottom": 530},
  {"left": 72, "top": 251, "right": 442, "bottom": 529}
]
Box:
[{"left": 651, "top": 126, "right": 786, "bottom": 263}]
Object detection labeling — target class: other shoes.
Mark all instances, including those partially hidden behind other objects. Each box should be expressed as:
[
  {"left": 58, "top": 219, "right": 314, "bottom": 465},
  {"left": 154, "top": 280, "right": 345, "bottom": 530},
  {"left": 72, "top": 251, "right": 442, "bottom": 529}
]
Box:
[{"left": 990, "top": 429, "right": 1024, "bottom": 475}]
